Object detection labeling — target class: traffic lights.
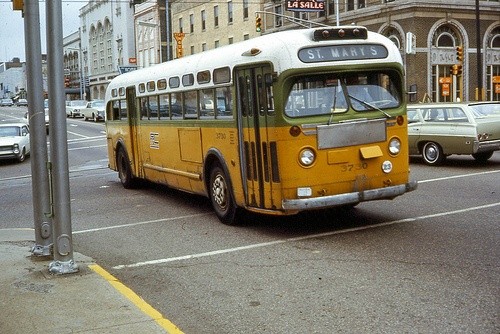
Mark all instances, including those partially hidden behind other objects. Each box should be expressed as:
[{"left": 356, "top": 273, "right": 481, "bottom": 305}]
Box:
[
  {"left": 256, "top": 17, "right": 262, "bottom": 32},
  {"left": 456, "top": 47, "right": 462, "bottom": 61},
  {"left": 450, "top": 65, "right": 462, "bottom": 75}
]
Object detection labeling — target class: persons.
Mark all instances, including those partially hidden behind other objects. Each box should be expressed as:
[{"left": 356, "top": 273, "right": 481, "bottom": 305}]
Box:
[
  {"left": 429, "top": 109, "right": 439, "bottom": 121},
  {"left": 338, "top": 76, "right": 373, "bottom": 109},
  {"left": 173, "top": 91, "right": 188, "bottom": 116}
]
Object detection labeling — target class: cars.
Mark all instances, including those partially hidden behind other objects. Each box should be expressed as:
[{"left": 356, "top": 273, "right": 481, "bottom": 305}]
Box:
[
  {"left": 0, "top": 123, "right": 30, "bottom": 162},
  {"left": 43, "top": 98, "right": 49, "bottom": 108},
  {"left": 0, "top": 99, "right": 12, "bottom": 107},
  {"left": 65, "top": 101, "right": 88, "bottom": 119},
  {"left": 404, "top": 102, "right": 500, "bottom": 167},
  {"left": 16, "top": 99, "right": 27, "bottom": 107},
  {"left": 80, "top": 100, "right": 105, "bottom": 123},
  {"left": 27, "top": 108, "right": 51, "bottom": 129}
]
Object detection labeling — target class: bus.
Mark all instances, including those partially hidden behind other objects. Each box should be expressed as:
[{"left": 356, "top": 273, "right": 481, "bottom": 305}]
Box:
[{"left": 105, "top": 27, "right": 419, "bottom": 225}]
